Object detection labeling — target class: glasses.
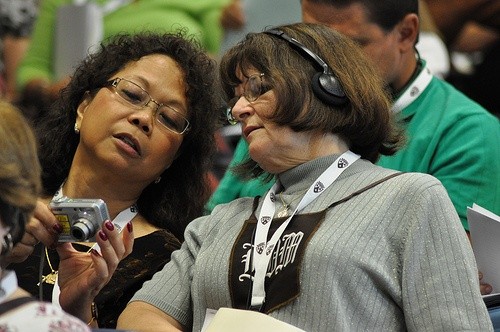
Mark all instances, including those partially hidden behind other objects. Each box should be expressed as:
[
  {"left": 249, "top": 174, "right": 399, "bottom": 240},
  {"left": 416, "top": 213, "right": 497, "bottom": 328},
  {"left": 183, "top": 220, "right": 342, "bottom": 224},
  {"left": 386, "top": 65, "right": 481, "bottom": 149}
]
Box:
[
  {"left": 226, "top": 73, "right": 264, "bottom": 126},
  {"left": 99, "top": 77, "right": 192, "bottom": 136}
]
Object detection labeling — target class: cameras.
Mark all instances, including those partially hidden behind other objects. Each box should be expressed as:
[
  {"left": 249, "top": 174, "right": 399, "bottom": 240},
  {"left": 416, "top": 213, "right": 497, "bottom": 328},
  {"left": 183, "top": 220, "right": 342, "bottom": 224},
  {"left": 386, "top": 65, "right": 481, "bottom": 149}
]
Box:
[{"left": 47, "top": 197, "right": 110, "bottom": 243}]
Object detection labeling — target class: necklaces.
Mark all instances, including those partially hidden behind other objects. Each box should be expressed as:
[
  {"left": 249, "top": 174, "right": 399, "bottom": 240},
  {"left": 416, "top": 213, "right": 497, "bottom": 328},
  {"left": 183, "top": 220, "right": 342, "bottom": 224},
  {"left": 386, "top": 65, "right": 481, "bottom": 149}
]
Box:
[
  {"left": 38, "top": 241, "right": 96, "bottom": 286},
  {"left": 277, "top": 186, "right": 310, "bottom": 218}
]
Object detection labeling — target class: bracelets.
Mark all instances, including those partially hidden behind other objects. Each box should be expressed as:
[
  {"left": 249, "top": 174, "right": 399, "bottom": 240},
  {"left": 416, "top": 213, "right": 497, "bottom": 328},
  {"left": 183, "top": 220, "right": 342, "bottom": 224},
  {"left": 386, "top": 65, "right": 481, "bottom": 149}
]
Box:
[{"left": 86, "top": 302, "right": 100, "bottom": 327}]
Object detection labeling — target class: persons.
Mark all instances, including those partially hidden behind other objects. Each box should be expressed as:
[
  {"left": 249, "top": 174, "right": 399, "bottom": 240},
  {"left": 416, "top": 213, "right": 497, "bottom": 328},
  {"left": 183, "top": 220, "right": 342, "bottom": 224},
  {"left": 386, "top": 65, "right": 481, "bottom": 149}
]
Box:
[
  {"left": 14, "top": 1, "right": 245, "bottom": 126},
  {"left": 6, "top": 28, "right": 226, "bottom": 332},
  {"left": 116, "top": 22, "right": 496, "bottom": 332},
  {"left": 0, "top": 99, "right": 92, "bottom": 332},
  {"left": 203, "top": 0, "right": 500, "bottom": 245}
]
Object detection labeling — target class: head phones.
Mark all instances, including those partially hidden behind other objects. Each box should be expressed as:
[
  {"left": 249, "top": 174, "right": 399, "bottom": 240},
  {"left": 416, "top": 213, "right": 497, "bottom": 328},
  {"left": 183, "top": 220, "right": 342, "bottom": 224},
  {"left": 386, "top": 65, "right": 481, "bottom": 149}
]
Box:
[{"left": 263, "top": 29, "right": 350, "bottom": 109}]
